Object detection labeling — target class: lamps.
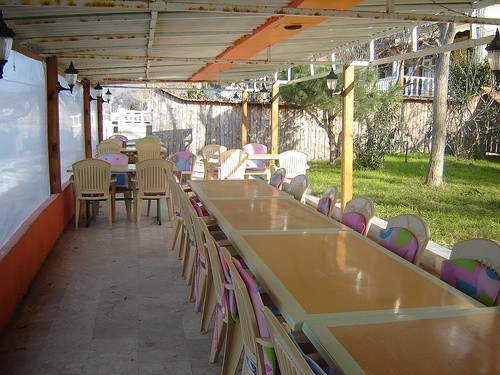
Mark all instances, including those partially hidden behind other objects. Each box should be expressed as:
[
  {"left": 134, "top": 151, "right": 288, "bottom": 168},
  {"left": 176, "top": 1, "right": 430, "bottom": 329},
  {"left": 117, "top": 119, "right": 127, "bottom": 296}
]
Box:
[
  {"left": 327, "top": 68, "right": 344, "bottom": 98},
  {"left": 260, "top": 85, "right": 272, "bottom": 107},
  {"left": 233, "top": 93, "right": 242, "bottom": 107},
  {"left": 0, "top": 10, "right": 15, "bottom": 79},
  {"left": 485, "top": 28, "right": 500, "bottom": 84},
  {"left": 102, "top": 89, "right": 112, "bottom": 104},
  {"left": 58, "top": 60, "right": 79, "bottom": 94},
  {"left": 90, "top": 82, "right": 104, "bottom": 102}
]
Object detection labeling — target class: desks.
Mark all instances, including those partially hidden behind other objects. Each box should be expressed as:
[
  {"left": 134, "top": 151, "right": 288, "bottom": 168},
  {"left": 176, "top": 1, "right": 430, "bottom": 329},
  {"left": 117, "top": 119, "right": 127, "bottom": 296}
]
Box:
[
  {"left": 302, "top": 306, "right": 500, "bottom": 375},
  {"left": 83, "top": 161, "right": 179, "bottom": 227},
  {"left": 225, "top": 230, "right": 488, "bottom": 333},
  {"left": 199, "top": 198, "right": 354, "bottom": 238},
  {"left": 186, "top": 178, "right": 294, "bottom": 200},
  {"left": 209, "top": 154, "right": 279, "bottom": 184}
]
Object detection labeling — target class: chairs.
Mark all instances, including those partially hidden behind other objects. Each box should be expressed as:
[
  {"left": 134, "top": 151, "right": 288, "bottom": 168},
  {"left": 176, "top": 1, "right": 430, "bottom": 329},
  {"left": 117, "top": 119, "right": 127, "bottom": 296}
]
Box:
[{"left": 69, "top": 134, "right": 500, "bottom": 375}]
